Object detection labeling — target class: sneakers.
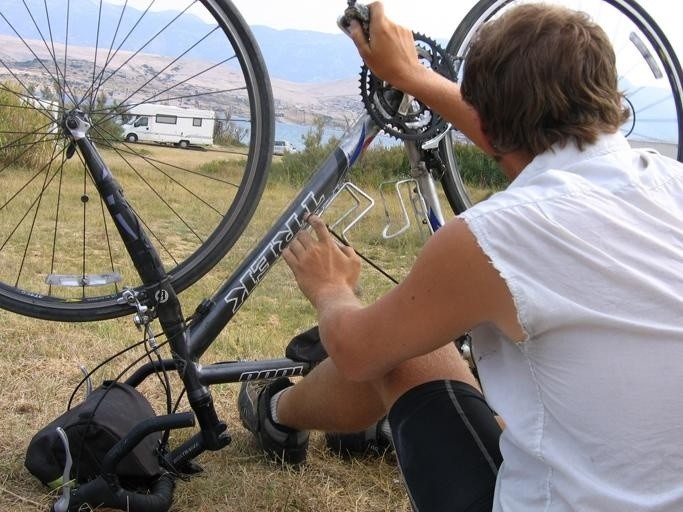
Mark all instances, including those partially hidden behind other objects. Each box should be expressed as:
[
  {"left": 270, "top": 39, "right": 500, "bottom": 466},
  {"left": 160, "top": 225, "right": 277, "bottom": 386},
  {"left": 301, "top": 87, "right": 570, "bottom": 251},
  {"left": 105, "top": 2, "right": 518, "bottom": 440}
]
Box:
[
  {"left": 237, "top": 376, "right": 310, "bottom": 471},
  {"left": 325, "top": 418, "right": 387, "bottom": 460}
]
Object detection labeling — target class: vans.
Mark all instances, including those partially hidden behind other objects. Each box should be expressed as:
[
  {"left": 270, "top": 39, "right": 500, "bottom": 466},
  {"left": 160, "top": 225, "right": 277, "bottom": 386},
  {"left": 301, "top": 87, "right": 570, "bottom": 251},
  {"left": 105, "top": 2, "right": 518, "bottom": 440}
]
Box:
[
  {"left": 113, "top": 103, "right": 214, "bottom": 149},
  {"left": 270, "top": 141, "right": 294, "bottom": 155}
]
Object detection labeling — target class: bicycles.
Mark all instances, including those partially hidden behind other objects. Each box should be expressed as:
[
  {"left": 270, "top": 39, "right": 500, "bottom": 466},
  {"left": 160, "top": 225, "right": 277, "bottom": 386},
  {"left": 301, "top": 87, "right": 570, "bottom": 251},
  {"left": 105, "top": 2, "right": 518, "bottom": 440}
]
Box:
[{"left": 0, "top": 0, "right": 683, "bottom": 512}]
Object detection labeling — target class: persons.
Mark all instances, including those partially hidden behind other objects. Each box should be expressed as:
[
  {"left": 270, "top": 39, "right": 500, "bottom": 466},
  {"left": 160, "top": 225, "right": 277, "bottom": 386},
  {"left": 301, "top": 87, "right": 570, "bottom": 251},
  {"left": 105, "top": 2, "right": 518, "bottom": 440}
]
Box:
[{"left": 239, "top": 1, "right": 683, "bottom": 512}]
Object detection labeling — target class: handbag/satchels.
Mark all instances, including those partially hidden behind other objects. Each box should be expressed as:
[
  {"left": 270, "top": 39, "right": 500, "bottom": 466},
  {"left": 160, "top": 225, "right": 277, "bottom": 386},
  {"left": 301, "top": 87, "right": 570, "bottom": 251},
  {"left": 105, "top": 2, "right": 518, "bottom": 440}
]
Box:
[{"left": 25, "top": 380, "right": 162, "bottom": 495}]
